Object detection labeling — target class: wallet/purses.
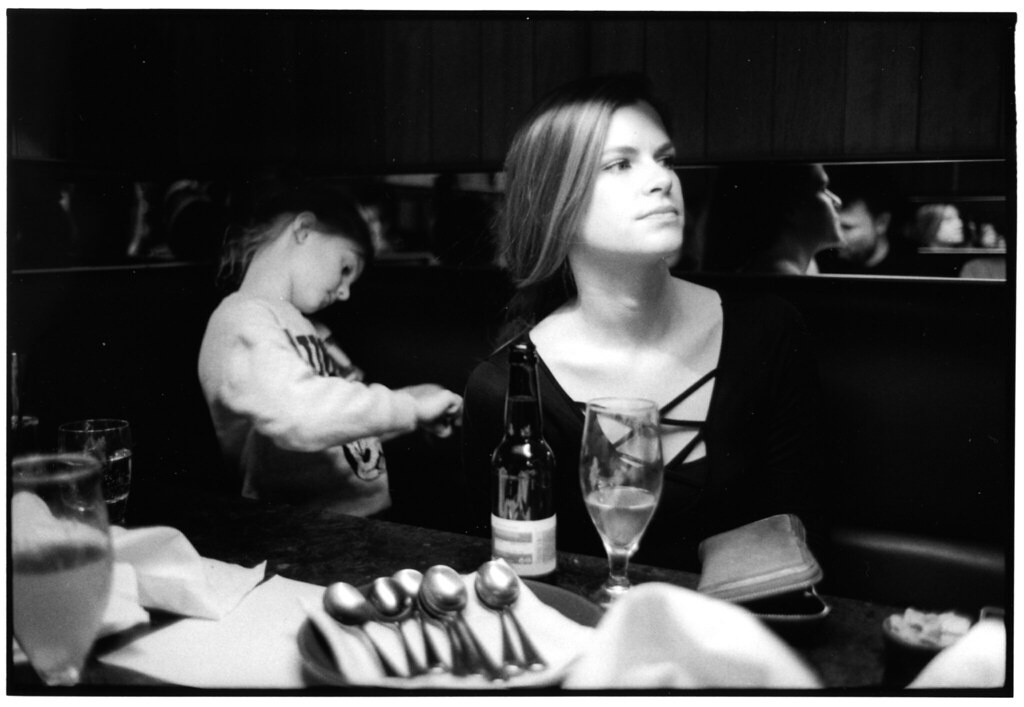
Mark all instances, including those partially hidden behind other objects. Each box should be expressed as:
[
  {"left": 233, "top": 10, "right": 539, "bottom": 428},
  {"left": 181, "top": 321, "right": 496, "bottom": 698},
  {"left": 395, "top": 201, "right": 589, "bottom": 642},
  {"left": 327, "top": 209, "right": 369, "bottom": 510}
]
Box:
[{"left": 698, "top": 510, "right": 831, "bottom": 632}]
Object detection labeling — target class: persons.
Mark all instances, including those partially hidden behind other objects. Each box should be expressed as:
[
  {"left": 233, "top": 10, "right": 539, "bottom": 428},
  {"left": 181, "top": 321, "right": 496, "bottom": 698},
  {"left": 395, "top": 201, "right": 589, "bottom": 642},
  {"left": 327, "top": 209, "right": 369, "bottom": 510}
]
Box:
[
  {"left": 462, "top": 69, "right": 838, "bottom": 588},
  {"left": 59, "top": 167, "right": 1008, "bottom": 525},
  {"left": 901, "top": 203, "right": 1006, "bottom": 272}
]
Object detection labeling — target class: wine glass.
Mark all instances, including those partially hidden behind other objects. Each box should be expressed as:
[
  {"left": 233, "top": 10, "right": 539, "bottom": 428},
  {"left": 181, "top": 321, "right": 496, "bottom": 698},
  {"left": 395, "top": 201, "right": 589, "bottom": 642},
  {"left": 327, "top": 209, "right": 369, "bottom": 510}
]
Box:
[{"left": 579, "top": 396, "right": 663, "bottom": 610}]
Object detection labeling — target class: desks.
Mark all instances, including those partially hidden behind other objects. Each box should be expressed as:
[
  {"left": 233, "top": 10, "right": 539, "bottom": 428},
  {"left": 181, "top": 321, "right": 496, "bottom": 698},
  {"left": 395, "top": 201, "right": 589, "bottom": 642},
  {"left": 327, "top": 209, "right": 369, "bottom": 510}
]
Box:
[{"left": 14, "top": 479, "right": 906, "bottom": 696}]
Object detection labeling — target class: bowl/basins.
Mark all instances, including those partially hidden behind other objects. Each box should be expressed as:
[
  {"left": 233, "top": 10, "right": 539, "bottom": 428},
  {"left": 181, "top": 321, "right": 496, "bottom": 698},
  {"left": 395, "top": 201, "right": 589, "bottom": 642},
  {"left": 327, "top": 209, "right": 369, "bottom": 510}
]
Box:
[{"left": 882, "top": 614, "right": 973, "bottom": 666}]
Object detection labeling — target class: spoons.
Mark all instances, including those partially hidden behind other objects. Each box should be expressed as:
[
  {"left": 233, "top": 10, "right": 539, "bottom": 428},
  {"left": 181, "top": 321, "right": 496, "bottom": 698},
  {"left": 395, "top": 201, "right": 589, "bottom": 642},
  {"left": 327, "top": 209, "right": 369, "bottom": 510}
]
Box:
[
  {"left": 366, "top": 564, "right": 512, "bottom": 683},
  {"left": 475, "top": 562, "right": 547, "bottom": 676},
  {"left": 323, "top": 581, "right": 403, "bottom": 678}
]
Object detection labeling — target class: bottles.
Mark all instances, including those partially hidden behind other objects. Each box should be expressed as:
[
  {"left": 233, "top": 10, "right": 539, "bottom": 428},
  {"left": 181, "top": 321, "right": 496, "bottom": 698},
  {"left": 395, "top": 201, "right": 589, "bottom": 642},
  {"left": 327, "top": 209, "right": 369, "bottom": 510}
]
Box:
[{"left": 491, "top": 343, "right": 557, "bottom": 584}]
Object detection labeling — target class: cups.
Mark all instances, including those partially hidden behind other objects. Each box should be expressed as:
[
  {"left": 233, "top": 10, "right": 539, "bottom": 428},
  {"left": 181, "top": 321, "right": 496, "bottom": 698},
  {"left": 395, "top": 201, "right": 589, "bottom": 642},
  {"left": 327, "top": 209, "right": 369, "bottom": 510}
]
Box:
[
  {"left": 10, "top": 414, "right": 43, "bottom": 458},
  {"left": 56, "top": 412, "right": 132, "bottom": 506},
  {"left": 12, "top": 454, "right": 116, "bottom": 688}
]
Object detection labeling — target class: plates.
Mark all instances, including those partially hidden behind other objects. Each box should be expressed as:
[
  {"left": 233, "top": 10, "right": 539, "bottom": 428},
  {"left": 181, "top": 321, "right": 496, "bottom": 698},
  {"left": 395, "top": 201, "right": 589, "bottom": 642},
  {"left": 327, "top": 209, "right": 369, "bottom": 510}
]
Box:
[{"left": 295, "top": 580, "right": 604, "bottom": 690}]
